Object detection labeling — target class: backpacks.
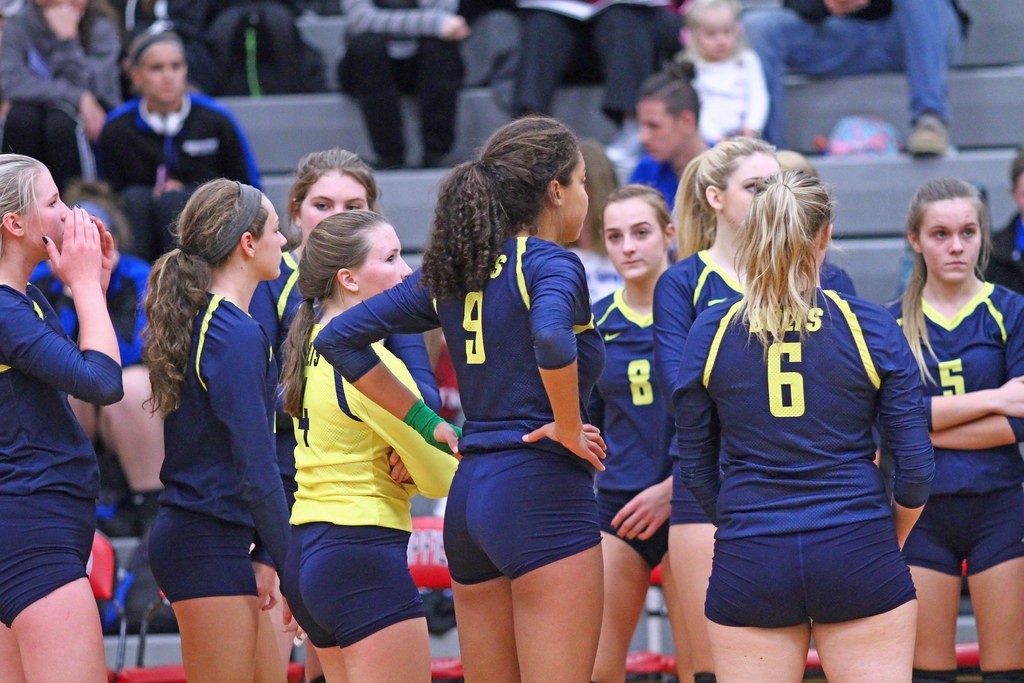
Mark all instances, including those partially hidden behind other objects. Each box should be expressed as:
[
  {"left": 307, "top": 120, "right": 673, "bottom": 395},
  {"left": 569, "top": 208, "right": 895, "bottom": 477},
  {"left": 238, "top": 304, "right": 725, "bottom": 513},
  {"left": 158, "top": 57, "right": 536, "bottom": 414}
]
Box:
[
  {"left": 104, "top": 525, "right": 179, "bottom": 634},
  {"left": 811, "top": 109, "right": 905, "bottom": 158},
  {"left": 207, "top": 2, "right": 323, "bottom": 97}
]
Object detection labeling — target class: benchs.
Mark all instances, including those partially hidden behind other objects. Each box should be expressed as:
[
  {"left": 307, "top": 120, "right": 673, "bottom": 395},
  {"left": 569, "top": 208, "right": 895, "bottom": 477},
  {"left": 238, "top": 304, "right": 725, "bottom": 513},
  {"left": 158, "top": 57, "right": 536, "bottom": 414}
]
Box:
[{"left": 211, "top": 0, "right": 1024, "bottom": 310}]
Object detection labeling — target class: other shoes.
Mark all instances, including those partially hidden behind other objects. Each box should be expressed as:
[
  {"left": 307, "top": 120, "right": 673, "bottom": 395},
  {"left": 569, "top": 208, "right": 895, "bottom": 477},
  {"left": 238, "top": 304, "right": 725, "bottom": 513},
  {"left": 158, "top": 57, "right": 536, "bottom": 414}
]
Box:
[
  {"left": 370, "top": 154, "right": 405, "bottom": 170},
  {"left": 423, "top": 151, "right": 454, "bottom": 167},
  {"left": 906, "top": 112, "right": 950, "bottom": 155},
  {"left": 604, "top": 124, "right": 640, "bottom": 169}
]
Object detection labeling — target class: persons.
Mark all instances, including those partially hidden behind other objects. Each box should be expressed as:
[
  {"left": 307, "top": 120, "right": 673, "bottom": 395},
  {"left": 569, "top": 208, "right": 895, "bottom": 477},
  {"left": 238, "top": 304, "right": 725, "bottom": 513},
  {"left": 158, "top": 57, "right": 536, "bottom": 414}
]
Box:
[
  {"left": 1, "top": 1, "right": 1024, "bottom": 683},
  {"left": 312, "top": 116, "right": 606, "bottom": 683}
]
